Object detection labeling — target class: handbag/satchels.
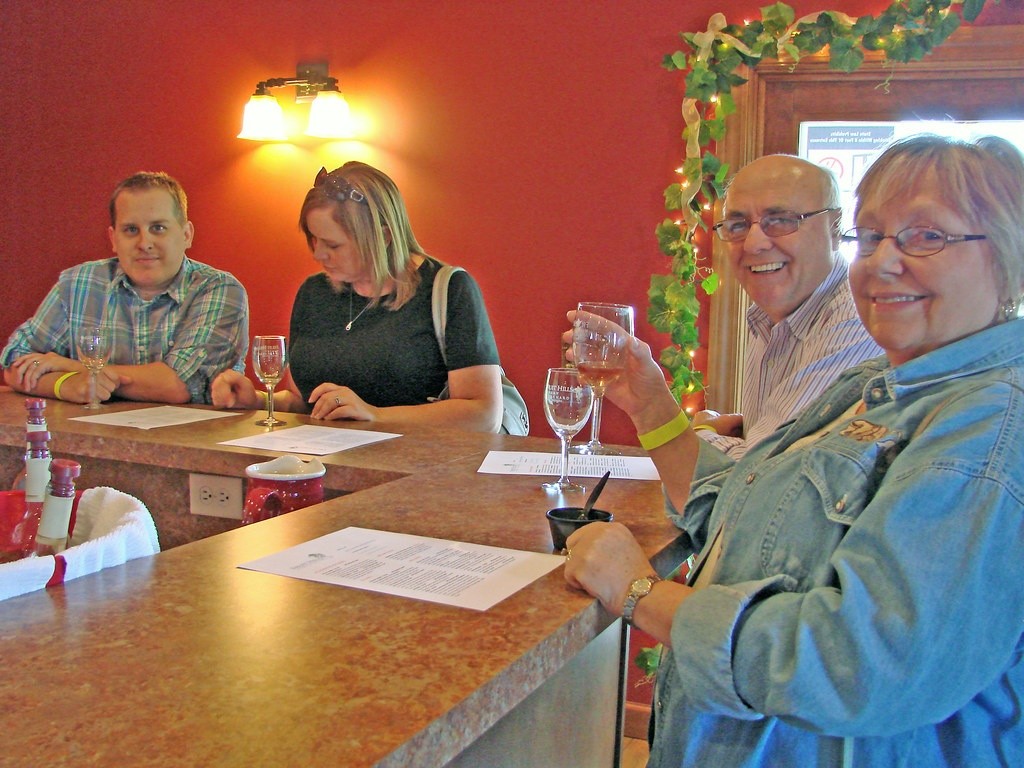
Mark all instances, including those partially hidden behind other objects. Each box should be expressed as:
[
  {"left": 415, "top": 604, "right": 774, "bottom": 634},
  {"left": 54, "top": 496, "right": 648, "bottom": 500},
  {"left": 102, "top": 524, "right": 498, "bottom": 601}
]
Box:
[{"left": 426, "top": 263, "right": 533, "bottom": 437}]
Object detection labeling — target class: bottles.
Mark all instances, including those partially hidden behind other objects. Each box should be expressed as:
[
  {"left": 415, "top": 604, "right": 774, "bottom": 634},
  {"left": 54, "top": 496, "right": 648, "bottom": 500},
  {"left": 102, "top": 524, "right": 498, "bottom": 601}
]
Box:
[{"left": 7, "top": 398, "right": 82, "bottom": 562}]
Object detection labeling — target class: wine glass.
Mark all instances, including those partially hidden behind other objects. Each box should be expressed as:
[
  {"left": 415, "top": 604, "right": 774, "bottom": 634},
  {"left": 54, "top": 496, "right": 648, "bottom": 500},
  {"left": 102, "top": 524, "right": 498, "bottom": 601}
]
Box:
[
  {"left": 74, "top": 326, "right": 113, "bottom": 411},
  {"left": 253, "top": 336, "right": 287, "bottom": 426},
  {"left": 571, "top": 301, "right": 633, "bottom": 457},
  {"left": 541, "top": 368, "right": 593, "bottom": 496}
]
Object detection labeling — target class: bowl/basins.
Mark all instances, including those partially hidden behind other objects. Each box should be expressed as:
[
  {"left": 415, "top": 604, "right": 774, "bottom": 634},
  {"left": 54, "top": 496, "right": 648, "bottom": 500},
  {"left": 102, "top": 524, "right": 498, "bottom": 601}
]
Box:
[{"left": 547, "top": 507, "right": 613, "bottom": 551}]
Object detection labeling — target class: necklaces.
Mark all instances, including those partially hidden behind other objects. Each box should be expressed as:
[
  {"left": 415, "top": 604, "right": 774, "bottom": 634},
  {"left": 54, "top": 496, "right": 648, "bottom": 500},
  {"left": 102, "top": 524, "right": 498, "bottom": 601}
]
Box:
[{"left": 346, "top": 283, "right": 382, "bottom": 331}]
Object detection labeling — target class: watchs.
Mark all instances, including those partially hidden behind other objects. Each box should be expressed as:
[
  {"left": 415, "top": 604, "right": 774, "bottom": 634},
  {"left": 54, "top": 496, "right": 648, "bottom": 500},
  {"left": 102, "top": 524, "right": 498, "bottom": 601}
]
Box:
[{"left": 621, "top": 574, "right": 663, "bottom": 625}]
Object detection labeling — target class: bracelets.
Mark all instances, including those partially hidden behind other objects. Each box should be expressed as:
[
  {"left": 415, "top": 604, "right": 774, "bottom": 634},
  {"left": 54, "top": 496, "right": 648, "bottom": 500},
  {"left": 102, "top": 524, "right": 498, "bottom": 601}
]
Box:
[
  {"left": 53, "top": 371, "right": 80, "bottom": 400},
  {"left": 623, "top": 408, "right": 690, "bottom": 452},
  {"left": 693, "top": 424, "right": 718, "bottom": 435}
]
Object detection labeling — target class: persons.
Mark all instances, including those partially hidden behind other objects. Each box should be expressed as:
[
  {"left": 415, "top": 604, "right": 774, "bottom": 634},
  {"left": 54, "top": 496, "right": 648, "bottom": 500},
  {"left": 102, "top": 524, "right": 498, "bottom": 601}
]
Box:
[
  {"left": 564, "top": 131, "right": 1024, "bottom": 768},
  {"left": 0, "top": 170, "right": 250, "bottom": 404},
  {"left": 211, "top": 161, "right": 504, "bottom": 434},
  {"left": 690, "top": 154, "right": 886, "bottom": 461}
]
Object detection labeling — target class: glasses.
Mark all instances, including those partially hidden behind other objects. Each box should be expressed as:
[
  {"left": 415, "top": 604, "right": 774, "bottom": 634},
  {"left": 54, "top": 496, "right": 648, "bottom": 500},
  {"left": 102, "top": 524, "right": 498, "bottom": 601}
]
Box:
[
  {"left": 314, "top": 167, "right": 367, "bottom": 207},
  {"left": 840, "top": 215, "right": 990, "bottom": 258},
  {"left": 712, "top": 201, "right": 843, "bottom": 243}
]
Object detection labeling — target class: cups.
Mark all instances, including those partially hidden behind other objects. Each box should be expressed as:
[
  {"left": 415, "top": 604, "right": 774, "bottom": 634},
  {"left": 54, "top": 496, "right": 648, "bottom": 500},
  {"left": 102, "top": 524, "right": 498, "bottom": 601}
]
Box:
[{"left": 241, "top": 455, "right": 326, "bottom": 527}]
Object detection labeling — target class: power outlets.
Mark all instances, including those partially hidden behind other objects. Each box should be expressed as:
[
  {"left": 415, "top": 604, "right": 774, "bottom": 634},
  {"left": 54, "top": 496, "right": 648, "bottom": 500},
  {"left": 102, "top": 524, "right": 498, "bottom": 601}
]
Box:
[{"left": 189, "top": 473, "right": 244, "bottom": 520}]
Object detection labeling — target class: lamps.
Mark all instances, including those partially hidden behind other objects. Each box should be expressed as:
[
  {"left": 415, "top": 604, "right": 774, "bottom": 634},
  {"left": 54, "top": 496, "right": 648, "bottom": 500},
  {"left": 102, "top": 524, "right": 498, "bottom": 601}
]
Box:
[{"left": 237, "top": 61, "right": 367, "bottom": 142}]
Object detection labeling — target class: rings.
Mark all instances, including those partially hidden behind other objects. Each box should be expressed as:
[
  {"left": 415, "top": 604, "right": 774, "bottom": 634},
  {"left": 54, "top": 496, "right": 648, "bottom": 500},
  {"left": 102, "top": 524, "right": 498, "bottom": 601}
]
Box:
[
  {"left": 33, "top": 360, "right": 40, "bottom": 367},
  {"left": 334, "top": 396, "right": 340, "bottom": 407},
  {"left": 566, "top": 549, "right": 572, "bottom": 563}
]
